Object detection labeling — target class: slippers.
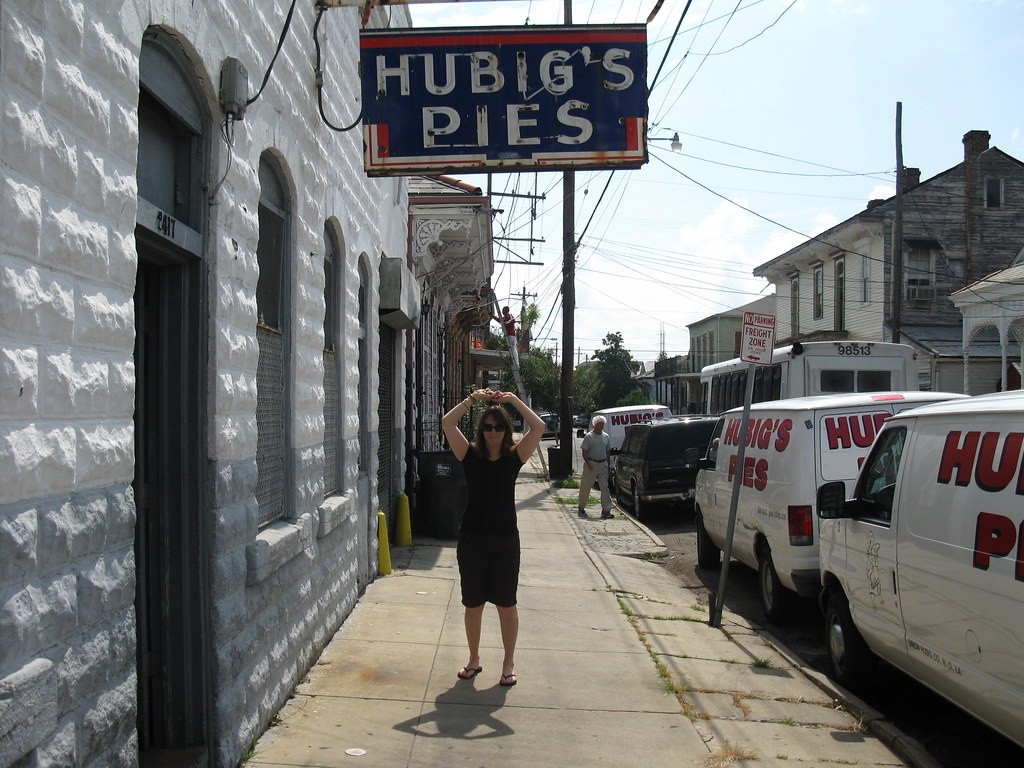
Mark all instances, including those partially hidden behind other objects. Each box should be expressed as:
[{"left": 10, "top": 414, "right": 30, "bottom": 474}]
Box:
[
  {"left": 500, "top": 674, "right": 517, "bottom": 686},
  {"left": 457, "top": 666, "right": 482, "bottom": 680}
]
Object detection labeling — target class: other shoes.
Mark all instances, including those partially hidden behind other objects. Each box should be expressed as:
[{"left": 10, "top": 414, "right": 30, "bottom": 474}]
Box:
[
  {"left": 577, "top": 507, "right": 587, "bottom": 517},
  {"left": 601, "top": 510, "right": 614, "bottom": 518}
]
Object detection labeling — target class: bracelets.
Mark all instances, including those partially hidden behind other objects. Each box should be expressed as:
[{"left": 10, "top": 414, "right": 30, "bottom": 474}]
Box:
[{"left": 461, "top": 394, "right": 476, "bottom": 412}]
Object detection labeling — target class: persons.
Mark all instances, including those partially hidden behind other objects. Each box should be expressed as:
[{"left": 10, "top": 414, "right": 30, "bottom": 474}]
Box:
[
  {"left": 491, "top": 306, "right": 520, "bottom": 371},
  {"left": 709, "top": 438, "right": 719, "bottom": 461},
  {"left": 578, "top": 415, "right": 614, "bottom": 519},
  {"left": 441, "top": 387, "right": 545, "bottom": 686}
]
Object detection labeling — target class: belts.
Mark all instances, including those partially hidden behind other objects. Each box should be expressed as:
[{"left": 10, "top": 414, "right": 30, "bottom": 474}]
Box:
[{"left": 588, "top": 457, "right": 606, "bottom": 463}]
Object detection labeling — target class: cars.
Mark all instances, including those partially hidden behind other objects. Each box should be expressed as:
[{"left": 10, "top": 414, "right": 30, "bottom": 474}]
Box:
[{"left": 573, "top": 415, "right": 589, "bottom": 428}]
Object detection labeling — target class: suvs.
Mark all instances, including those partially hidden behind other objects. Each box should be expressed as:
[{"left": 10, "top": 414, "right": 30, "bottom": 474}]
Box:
[{"left": 538, "top": 414, "right": 560, "bottom": 439}]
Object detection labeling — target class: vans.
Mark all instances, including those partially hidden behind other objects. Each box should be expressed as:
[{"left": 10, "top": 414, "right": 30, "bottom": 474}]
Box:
[
  {"left": 577, "top": 405, "right": 674, "bottom": 490},
  {"left": 812, "top": 390, "right": 1024, "bottom": 754},
  {"left": 610, "top": 416, "right": 722, "bottom": 519},
  {"left": 686, "top": 389, "right": 972, "bottom": 628}
]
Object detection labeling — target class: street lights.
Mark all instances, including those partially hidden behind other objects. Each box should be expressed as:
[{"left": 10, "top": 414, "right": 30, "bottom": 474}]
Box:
[
  {"left": 524, "top": 337, "right": 558, "bottom": 409},
  {"left": 559, "top": 133, "right": 683, "bottom": 478}
]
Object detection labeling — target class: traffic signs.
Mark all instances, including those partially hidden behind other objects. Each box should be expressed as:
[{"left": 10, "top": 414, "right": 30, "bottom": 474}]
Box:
[{"left": 741, "top": 313, "right": 774, "bottom": 364}]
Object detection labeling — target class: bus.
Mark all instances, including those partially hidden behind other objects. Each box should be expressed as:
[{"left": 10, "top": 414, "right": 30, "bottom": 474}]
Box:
[{"left": 687, "top": 339, "right": 918, "bottom": 414}]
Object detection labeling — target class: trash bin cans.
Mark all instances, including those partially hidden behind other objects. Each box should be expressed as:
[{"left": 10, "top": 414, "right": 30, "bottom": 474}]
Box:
[
  {"left": 547, "top": 444, "right": 561, "bottom": 480},
  {"left": 415, "top": 450, "right": 465, "bottom": 540}
]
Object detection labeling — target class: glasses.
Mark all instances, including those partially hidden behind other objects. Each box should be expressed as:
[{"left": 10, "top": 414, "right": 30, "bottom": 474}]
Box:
[{"left": 481, "top": 424, "right": 505, "bottom": 433}]
